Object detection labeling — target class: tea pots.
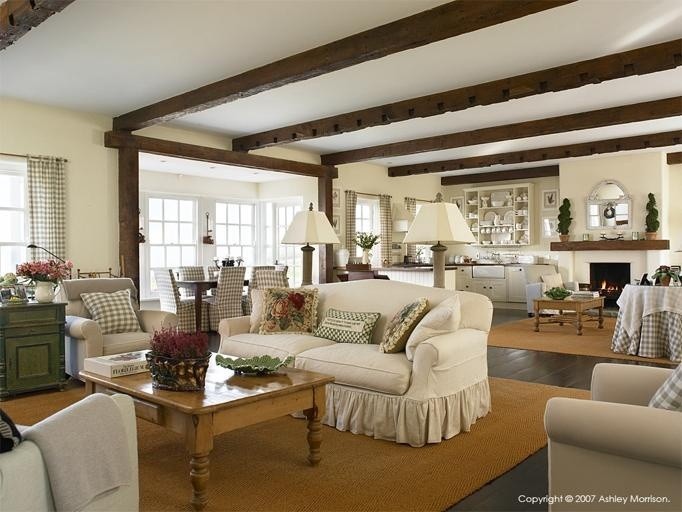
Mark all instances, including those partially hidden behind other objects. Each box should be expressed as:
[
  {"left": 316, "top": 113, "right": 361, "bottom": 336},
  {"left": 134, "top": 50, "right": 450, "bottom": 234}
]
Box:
[{"left": 493, "top": 214, "right": 501, "bottom": 225}]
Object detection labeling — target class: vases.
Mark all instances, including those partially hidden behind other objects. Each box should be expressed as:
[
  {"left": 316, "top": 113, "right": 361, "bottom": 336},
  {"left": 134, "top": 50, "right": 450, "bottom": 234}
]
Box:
[
  {"left": 334, "top": 245, "right": 350, "bottom": 266},
  {"left": 34, "top": 281, "right": 57, "bottom": 303},
  {"left": 145, "top": 351, "right": 213, "bottom": 394}
]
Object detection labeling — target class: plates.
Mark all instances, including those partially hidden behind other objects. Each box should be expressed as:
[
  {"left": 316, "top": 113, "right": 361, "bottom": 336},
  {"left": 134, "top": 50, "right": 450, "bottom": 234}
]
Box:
[
  {"left": 215, "top": 355, "right": 294, "bottom": 375},
  {"left": 480, "top": 211, "right": 497, "bottom": 225},
  {"left": 500, "top": 211, "right": 515, "bottom": 224}
]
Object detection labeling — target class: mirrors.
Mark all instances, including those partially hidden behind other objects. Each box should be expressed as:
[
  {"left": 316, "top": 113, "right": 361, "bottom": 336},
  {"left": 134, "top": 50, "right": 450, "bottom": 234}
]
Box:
[{"left": 585, "top": 179, "right": 634, "bottom": 231}]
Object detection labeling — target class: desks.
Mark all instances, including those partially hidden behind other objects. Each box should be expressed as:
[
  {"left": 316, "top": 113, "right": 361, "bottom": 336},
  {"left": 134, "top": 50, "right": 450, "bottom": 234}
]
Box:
[{"left": 609, "top": 284, "right": 682, "bottom": 361}]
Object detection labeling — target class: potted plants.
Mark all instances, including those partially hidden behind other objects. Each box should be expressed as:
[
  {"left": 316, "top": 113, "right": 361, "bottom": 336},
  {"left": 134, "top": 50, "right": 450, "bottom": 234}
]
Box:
[
  {"left": 554, "top": 198, "right": 574, "bottom": 242},
  {"left": 351, "top": 232, "right": 381, "bottom": 264},
  {"left": 644, "top": 192, "right": 660, "bottom": 240},
  {"left": 652, "top": 265, "right": 677, "bottom": 286}
]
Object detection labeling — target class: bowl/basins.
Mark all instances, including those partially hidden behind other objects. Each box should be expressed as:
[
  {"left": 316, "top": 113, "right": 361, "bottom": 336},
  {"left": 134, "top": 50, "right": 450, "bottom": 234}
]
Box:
[{"left": 481, "top": 240, "right": 515, "bottom": 245}]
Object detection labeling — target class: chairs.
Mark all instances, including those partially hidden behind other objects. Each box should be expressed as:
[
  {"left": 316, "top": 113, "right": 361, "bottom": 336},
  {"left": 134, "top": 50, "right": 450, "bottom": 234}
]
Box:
[
  {"left": 0, "top": 390, "right": 139, "bottom": 511},
  {"left": 543, "top": 358, "right": 682, "bottom": 512},
  {"left": 54, "top": 277, "right": 180, "bottom": 386},
  {"left": 525, "top": 280, "right": 580, "bottom": 319},
  {"left": 153, "top": 266, "right": 291, "bottom": 335}
]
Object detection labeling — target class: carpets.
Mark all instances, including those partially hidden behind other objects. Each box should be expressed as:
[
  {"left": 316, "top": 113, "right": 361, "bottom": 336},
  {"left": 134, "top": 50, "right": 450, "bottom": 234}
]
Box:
[
  {"left": 488, "top": 315, "right": 682, "bottom": 366},
  {"left": 0, "top": 374, "right": 594, "bottom": 512}
]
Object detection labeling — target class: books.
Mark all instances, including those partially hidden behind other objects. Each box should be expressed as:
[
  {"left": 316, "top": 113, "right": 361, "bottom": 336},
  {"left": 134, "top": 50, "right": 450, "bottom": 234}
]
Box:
[
  {"left": 572, "top": 291, "right": 599, "bottom": 295},
  {"left": 570, "top": 294, "right": 599, "bottom": 298}
]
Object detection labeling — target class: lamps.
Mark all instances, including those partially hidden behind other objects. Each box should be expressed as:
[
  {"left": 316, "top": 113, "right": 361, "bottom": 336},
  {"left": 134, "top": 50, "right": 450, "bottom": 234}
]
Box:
[
  {"left": 280, "top": 203, "right": 341, "bottom": 286},
  {"left": 596, "top": 182, "right": 625, "bottom": 229},
  {"left": 402, "top": 191, "right": 477, "bottom": 289}
]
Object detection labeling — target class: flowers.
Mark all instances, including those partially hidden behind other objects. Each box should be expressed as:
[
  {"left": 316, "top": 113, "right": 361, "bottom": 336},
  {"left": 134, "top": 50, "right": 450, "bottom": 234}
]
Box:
[
  {"left": 148, "top": 323, "right": 211, "bottom": 357},
  {"left": 13, "top": 260, "right": 74, "bottom": 283}
]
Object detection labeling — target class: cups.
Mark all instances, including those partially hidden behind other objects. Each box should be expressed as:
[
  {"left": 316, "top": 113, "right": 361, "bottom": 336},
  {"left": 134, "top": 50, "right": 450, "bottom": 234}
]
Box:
[
  {"left": 479, "top": 227, "right": 514, "bottom": 235},
  {"left": 632, "top": 232, "right": 640, "bottom": 241},
  {"left": 449, "top": 256, "right": 466, "bottom": 264},
  {"left": 583, "top": 234, "right": 590, "bottom": 241}
]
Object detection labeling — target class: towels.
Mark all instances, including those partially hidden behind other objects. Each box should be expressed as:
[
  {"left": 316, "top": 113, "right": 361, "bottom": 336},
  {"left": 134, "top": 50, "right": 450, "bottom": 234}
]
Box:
[{"left": 18, "top": 392, "right": 134, "bottom": 512}]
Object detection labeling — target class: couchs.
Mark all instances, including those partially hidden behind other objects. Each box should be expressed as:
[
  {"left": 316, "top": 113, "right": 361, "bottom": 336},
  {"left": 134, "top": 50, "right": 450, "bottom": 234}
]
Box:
[{"left": 216, "top": 276, "right": 497, "bottom": 448}]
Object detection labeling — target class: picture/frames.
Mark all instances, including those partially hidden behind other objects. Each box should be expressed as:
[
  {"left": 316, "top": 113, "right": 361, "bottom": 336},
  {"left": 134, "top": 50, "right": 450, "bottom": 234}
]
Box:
[
  {"left": 541, "top": 216, "right": 558, "bottom": 239},
  {"left": 638, "top": 272, "right": 650, "bottom": 286},
  {"left": 451, "top": 196, "right": 464, "bottom": 214},
  {"left": 542, "top": 189, "right": 559, "bottom": 211}
]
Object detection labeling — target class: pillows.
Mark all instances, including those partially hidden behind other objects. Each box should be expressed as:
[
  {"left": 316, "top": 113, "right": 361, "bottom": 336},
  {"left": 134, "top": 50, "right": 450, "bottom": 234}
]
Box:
[
  {"left": 539, "top": 272, "right": 565, "bottom": 291},
  {"left": 648, "top": 358, "right": 682, "bottom": 411},
  {"left": 258, "top": 285, "right": 321, "bottom": 336},
  {"left": 380, "top": 296, "right": 432, "bottom": 356},
  {"left": 313, "top": 307, "right": 381, "bottom": 344},
  {"left": 404, "top": 295, "right": 464, "bottom": 362},
  {"left": 79, "top": 288, "right": 144, "bottom": 336}
]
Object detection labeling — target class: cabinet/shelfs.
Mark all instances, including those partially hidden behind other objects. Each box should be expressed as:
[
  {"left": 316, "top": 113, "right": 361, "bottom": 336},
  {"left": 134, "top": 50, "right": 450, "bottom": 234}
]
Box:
[
  {"left": 462, "top": 187, "right": 479, "bottom": 246},
  {"left": 505, "top": 265, "right": 555, "bottom": 310},
  {"left": 472, "top": 265, "right": 506, "bottom": 309},
  {"left": 514, "top": 183, "right": 534, "bottom": 245},
  {"left": 478, "top": 186, "right": 514, "bottom": 247},
  {"left": 0, "top": 302, "right": 68, "bottom": 402},
  {"left": 456, "top": 265, "right": 472, "bottom": 292}
]
